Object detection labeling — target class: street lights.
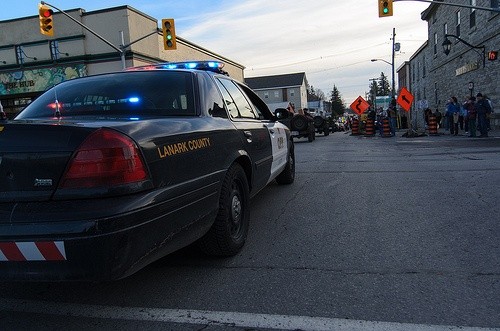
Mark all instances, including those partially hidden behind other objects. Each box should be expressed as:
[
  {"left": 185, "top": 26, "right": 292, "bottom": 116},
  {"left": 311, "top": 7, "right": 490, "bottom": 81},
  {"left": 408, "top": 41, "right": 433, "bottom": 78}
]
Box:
[{"left": 371, "top": 58, "right": 397, "bottom": 132}]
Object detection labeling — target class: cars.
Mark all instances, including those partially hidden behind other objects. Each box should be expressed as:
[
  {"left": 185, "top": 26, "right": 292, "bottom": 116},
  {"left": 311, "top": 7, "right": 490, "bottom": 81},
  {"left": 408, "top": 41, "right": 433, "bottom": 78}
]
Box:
[{"left": 1, "top": 60, "right": 297, "bottom": 285}]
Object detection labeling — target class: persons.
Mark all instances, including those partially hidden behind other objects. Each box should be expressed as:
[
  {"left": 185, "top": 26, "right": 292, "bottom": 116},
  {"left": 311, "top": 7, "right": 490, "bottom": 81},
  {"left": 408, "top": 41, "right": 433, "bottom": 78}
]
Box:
[
  {"left": 288, "top": 102, "right": 374, "bottom": 132},
  {"left": 434, "top": 108, "right": 442, "bottom": 130},
  {"left": 445, "top": 93, "right": 494, "bottom": 138},
  {"left": 376, "top": 96, "right": 396, "bottom": 136}
]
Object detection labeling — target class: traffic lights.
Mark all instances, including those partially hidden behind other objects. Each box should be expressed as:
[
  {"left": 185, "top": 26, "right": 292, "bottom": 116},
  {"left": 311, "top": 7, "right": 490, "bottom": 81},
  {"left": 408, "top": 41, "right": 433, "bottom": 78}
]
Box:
[
  {"left": 378, "top": 0, "right": 393, "bottom": 17},
  {"left": 162, "top": 18, "right": 177, "bottom": 50},
  {"left": 38, "top": 3, "right": 54, "bottom": 36}
]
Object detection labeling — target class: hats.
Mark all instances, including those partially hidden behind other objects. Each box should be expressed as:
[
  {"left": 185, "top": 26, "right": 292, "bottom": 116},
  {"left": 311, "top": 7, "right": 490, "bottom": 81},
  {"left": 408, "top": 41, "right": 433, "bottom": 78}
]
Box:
[
  {"left": 476, "top": 93, "right": 483, "bottom": 97},
  {"left": 303, "top": 108, "right": 309, "bottom": 111}
]
